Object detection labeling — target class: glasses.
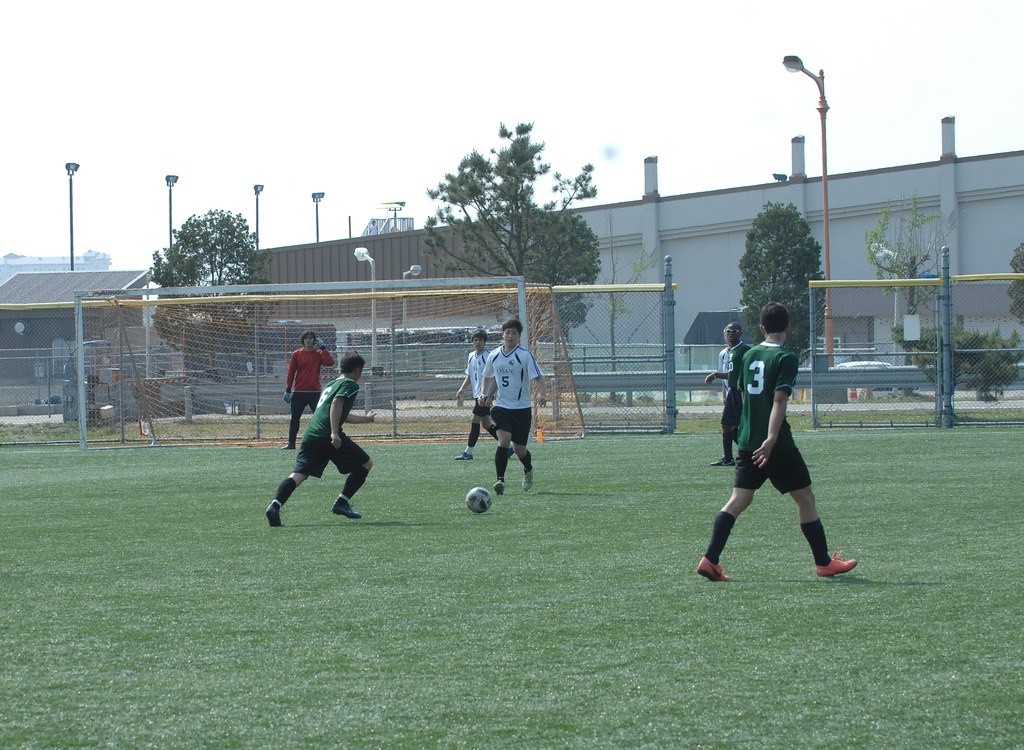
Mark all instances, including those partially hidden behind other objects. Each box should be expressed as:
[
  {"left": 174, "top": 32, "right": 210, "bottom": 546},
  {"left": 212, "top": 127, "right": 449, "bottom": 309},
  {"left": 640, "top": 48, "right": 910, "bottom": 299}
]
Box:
[{"left": 726, "top": 329, "right": 740, "bottom": 333}]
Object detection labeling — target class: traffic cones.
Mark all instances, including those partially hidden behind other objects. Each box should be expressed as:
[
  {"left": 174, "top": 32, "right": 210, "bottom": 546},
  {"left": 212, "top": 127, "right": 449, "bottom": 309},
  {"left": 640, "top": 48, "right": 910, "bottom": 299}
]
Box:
[{"left": 850, "top": 387, "right": 857, "bottom": 400}]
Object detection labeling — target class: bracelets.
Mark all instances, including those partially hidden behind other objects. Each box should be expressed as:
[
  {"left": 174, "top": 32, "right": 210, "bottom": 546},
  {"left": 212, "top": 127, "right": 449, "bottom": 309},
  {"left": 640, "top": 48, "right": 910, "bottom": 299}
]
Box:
[{"left": 712, "top": 373, "right": 716, "bottom": 379}]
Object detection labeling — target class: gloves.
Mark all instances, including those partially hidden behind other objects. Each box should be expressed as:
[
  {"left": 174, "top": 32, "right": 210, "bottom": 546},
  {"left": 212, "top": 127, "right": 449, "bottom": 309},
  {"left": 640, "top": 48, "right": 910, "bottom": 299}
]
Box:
[
  {"left": 283, "top": 392, "right": 292, "bottom": 403},
  {"left": 314, "top": 338, "right": 325, "bottom": 348}
]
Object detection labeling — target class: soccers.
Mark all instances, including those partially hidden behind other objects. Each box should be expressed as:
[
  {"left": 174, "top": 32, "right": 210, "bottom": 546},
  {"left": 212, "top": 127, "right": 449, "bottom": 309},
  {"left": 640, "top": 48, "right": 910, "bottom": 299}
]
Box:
[{"left": 465, "top": 486, "right": 493, "bottom": 513}]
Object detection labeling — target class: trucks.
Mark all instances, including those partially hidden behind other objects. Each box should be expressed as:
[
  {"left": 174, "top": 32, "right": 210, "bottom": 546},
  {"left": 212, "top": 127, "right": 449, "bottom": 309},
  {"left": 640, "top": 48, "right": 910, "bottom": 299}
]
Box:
[{"left": 63, "top": 340, "right": 171, "bottom": 384}]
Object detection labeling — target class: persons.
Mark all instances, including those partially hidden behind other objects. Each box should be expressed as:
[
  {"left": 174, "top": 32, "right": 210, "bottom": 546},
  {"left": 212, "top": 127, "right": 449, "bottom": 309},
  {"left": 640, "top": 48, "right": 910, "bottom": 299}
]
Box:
[
  {"left": 280, "top": 331, "right": 335, "bottom": 450},
  {"left": 697, "top": 301, "right": 858, "bottom": 581},
  {"left": 479, "top": 320, "right": 547, "bottom": 495},
  {"left": 266, "top": 352, "right": 377, "bottom": 527},
  {"left": 705, "top": 322, "right": 751, "bottom": 465},
  {"left": 454, "top": 330, "right": 514, "bottom": 461},
  {"left": 717, "top": 329, "right": 732, "bottom": 405},
  {"left": 370, "top": 220, "right": 378, "bottom": 235}
]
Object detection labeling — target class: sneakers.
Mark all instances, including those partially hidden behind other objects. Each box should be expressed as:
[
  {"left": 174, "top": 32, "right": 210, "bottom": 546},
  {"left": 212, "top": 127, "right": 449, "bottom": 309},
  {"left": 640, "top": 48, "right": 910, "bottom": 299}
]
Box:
[
  {"left": 711, "top": 455, "right": 736, "bottom": 466},
  {"left": 522, "top": 468, "right": 534, "bottom": 492},
  {"left": 493, "top": 480, "right": 505, "bottom": 495},
  {"left": 455, "top": 452, "right": 473, "bottom": 460},
  {"left": 265, "top": 504, "right": 285, "bottom": 527},
  {"left": 508, "top": 446, "right": 514, "bottom": 458},
  {"left": 815, "top": 548, "right": 857, "bottom": 577},
  {"left": 331, "top": 503, "right": 362, "bottom": 518},
  {"left": 697, "top": 556, "right": 731, "bottom": 581}
]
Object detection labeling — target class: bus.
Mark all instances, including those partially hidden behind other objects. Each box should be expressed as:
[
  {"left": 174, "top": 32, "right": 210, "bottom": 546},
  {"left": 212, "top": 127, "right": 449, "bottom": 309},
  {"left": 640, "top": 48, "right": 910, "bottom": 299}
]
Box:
[
  {"left": 171, "top": 320, "right": 337, "bottom": 378},
  {"left": 347, "top": 325, "right": 504, "bottom": 374}
]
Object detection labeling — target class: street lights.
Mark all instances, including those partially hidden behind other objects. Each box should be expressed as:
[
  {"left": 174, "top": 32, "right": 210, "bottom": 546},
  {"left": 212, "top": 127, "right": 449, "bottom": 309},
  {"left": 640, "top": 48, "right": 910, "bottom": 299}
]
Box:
[
  {"left": 870, "top": 242, "right": 900, "bottom": 394},
  {"left": 66, "top": 163, "right": 79, "bottom": 269},
  {"left": 166, "top": 175, "right": 180, "bottom": 248},
  {"left": 311, "top": 193, "right": 324, "bottom": 243},
  {"left": 146, "top": 274, "right": 156, "bottom": 378},
  {"left": 254, "top": 185, "right": 264, "bottom": 251},
  {"left": 354, "top": 247, "right": 376, "bottom": 373},
  {"left": 782, "top": 55, "right": 834, "bottom": 369},
  {"left": 402, "top": 265, "right": 422, "bottom": 371}
]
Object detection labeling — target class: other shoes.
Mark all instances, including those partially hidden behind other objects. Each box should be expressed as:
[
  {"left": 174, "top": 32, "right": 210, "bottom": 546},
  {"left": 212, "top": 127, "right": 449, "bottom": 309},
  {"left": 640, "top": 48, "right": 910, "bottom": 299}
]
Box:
[{"left": 281, "top": 444, "right": 295, "bottom": 450}]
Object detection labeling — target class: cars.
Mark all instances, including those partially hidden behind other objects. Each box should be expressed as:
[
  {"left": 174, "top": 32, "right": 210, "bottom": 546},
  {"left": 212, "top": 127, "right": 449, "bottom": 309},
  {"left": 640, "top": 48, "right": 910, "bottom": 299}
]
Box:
[{"left": 838, "top": 362, "right": 892, "bottom": 368}]
